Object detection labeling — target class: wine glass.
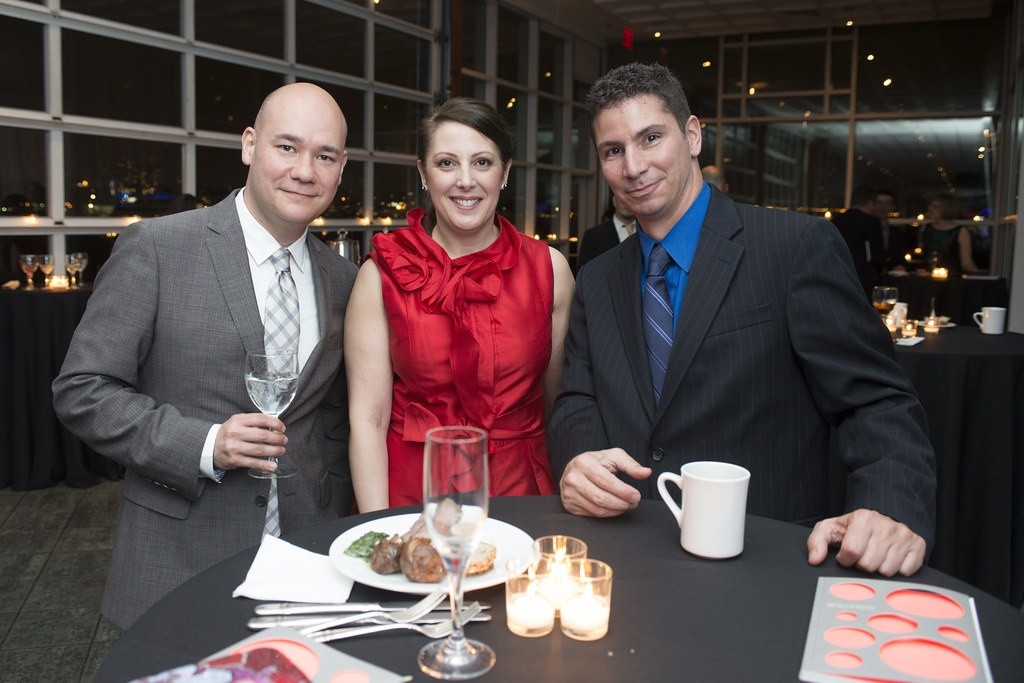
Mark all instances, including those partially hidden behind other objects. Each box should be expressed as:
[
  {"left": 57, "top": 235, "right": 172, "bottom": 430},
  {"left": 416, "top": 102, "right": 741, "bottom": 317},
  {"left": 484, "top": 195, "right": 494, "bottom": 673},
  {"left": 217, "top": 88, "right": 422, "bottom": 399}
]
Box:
[
  {"left": 243, "top": 348, "right": 298, "bottom": 480},
  {"left": 65, "top": 251, "right": 87, "bottom": 290},
  {"left": 18, "top": 254, "right": 53, "bottom": 290},
  {"left": 868, "top": 284, "right": 899, "bottom": 328},
  {"left": 416, "top": 427, "right": 489, "bottom": 681}
]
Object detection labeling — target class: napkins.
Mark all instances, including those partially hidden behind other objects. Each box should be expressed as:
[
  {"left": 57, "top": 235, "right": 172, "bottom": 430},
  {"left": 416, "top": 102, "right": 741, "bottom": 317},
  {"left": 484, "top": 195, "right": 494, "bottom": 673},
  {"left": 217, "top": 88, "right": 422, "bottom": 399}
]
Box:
[
  {"left": 894, "top": 336, "right": 926, "bottom": 346},
  {"left": 231, "top": 533, "right": 355, "bottom": 603}
]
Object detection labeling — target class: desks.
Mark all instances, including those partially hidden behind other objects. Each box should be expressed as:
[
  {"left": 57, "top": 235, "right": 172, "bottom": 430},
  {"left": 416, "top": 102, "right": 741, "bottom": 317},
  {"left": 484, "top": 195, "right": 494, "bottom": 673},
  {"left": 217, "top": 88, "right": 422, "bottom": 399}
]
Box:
[
  {"left": 891, "top": 325, "right": 1024, "bottom": 607},
  {"left": 1, "top": 285, "right": 124, "bottom": 488},
  {"left": 885, "top": 271, "right": 1008, "bottom": 325},
  {"left": 95, "top": 494, "right": 1024, "bottom": 683}
]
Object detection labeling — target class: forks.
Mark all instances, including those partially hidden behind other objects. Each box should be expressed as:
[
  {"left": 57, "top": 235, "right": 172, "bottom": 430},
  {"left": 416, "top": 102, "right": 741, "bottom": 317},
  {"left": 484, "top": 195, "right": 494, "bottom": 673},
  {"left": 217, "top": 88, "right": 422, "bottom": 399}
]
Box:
[{"left": 302, "top": 584, "right": 481, "bottom": 643}]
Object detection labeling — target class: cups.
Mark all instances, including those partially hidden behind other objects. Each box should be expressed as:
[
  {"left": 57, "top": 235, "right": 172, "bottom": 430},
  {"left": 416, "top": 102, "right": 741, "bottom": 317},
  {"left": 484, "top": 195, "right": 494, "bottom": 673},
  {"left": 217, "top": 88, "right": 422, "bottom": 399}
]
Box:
[
  {"left": 900, "top": 319, "right": 919, "bottom": 340},
  {"left": 327, "top": 239, "right": 360, "bottom": 268},
  {"left": 924, "top": 317, "right": 940, "bottom": 334},
  {"left": 883, "top": 315, "right": 898, "bottom": 331},
  {"left": 655, "top": 461, "right": 751, "bottom": 561},
  {"left": 973, "top": 306, "right": 1007, "bottom": 335},
  {"left": 505, "top": 535, "right": 614, "bottom": 641}
]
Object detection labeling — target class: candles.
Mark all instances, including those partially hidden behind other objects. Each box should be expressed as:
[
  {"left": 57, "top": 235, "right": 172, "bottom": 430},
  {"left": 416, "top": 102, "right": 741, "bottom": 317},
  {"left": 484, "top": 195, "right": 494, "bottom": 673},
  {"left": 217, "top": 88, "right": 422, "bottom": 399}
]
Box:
[
  {"left": 500, "top": 535, "right": 613, "bottom": 640},
  {"left": 894, "top": 313, "right": 945, "bottom": 339}
]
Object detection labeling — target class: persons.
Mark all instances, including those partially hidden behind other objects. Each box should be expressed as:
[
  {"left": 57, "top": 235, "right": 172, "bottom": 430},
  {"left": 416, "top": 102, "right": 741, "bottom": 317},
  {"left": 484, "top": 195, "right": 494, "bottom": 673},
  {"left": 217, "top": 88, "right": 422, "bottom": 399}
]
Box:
[
  {"left": 831, "top": 184, "right": 885, "bottom": 305},
  {"left": 343, "top": 96, "right": 574, "bottom": 514},
  {"left": 917, "top": 192, "right": 979, "bottom": 326},
  {"left": 579, "top": 185, "right": 639, "bottom": 269},
  {"left": 546, "top": 59, "right": 937, "bottom": 577},
  {"left": 51, "top": 82, "right": 360, "bottom": 632},
  {"left": 167, "top": 194, "right": 205, "bottom": 215},
  {"left": 702, "top": 165, "right": 728, "bottom": 196}
]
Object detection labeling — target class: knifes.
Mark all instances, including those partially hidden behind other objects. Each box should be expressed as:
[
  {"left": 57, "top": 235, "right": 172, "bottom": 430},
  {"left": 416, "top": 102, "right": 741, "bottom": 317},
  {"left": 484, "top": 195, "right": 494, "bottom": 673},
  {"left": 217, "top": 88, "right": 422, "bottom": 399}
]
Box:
[
  {"left": 248, "top": 611, "right": 492, "bottom": 632},
  {"left": 255, "top": 600, "right": 493, "bottom": 617}
]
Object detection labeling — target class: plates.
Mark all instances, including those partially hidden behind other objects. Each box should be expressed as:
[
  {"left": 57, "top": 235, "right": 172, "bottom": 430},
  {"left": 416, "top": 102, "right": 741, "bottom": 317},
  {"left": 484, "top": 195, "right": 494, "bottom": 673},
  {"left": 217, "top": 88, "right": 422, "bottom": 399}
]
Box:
[
  {"left": 918, "top": 320, "right": 957, "bottom": 327},
  {"left": 328, "top": 510, "right": 541, "bottom": 594}
]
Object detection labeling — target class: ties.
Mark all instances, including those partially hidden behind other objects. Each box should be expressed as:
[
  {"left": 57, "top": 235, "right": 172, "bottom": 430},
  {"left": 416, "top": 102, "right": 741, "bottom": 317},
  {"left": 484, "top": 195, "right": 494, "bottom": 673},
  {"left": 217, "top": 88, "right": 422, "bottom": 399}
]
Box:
[
  {"left": 263, "top": 249, "right": 300, "bottom": 539},
  {"left": 642, "top": 245, "right": 674, "bottom": 407},
  {"left": 624, "top": 224, "right": 634, "bottom": 236}
]
